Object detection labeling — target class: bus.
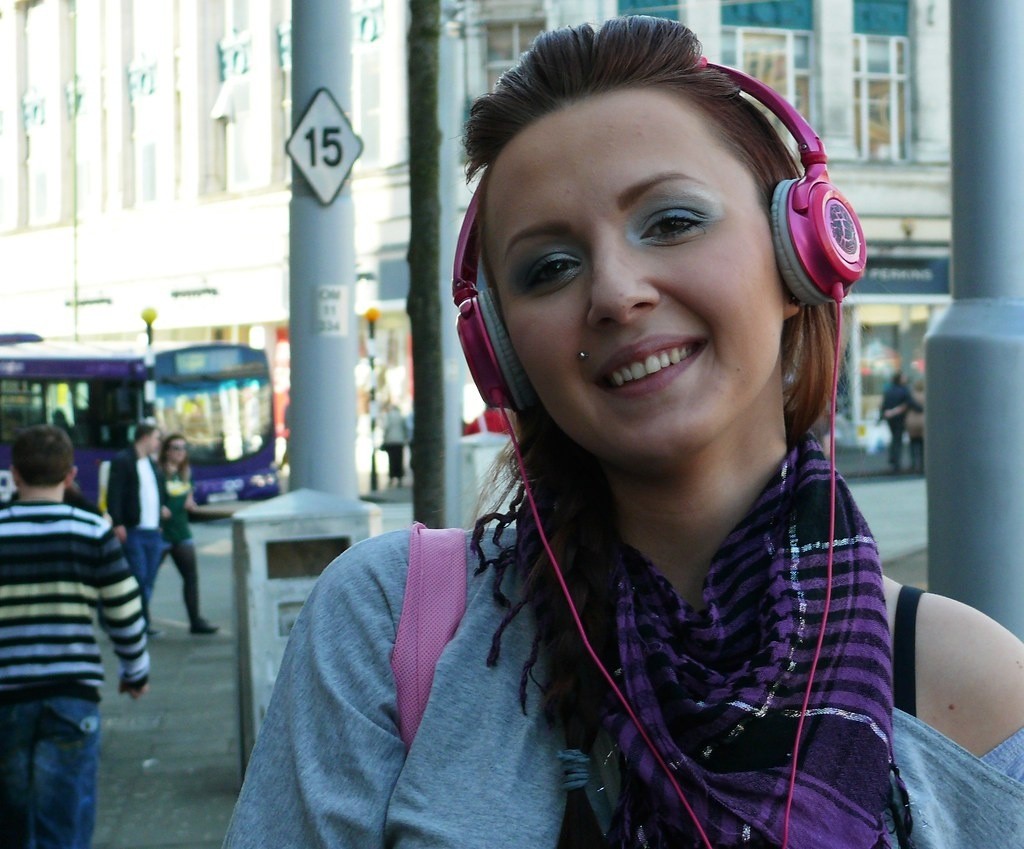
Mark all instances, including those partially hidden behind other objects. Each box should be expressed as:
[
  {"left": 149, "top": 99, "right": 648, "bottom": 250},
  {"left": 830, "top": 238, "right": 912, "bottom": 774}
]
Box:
[{"left": 0, "top": 333, "right": 279, "bottom": 517}]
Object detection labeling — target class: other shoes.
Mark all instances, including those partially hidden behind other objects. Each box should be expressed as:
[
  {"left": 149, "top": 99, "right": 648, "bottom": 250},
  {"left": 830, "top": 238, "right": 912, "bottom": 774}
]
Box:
[{"left": 190, "top": 617, "right": 220, "bottom": 633}]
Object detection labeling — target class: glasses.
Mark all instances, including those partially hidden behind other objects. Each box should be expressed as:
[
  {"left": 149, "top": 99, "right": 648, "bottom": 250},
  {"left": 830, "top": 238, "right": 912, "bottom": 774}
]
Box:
[{"left": 168, "top": 445, "right": 188, "bottom": 451}]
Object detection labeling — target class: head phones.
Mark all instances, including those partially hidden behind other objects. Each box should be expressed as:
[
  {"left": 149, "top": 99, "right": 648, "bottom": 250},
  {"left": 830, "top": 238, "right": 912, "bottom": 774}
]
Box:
[{"left": 452, "top": 61, "right": 867, "bottom": 414}]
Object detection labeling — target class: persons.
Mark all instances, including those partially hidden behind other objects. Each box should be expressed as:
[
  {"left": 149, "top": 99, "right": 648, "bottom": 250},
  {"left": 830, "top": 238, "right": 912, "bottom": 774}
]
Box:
[
  {"left": 464, "top": 404, "right": 511, "bottom": 434},
  {"left": 222, "top": 17, "right": 1024, "bottom": 849},
  {"left": 0, "top": 426, "right": 151, "bottom": 848},
  {"left": 885, "top": 380, "right": 924, "bottom": 472},
  {"left": 106, "top": 424, "right": 162, "bottom": 636},
  {"left": 158, "top": 434, "right": 219, "bottom": 634},
  {"left": 381, "top": 400, "right": 415, "bottom": 487},
  {"left": 879, "top": 372, "right": 924, "bottom": 472}
]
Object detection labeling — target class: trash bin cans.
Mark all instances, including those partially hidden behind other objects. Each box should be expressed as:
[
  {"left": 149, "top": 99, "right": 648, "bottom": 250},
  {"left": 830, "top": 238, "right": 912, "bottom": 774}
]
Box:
[
  {"left": 459, "top": 431, "right": 518, "bottom": 531},
  {"left": 233, "top": 486, "right": 384, "bottom": 788}
]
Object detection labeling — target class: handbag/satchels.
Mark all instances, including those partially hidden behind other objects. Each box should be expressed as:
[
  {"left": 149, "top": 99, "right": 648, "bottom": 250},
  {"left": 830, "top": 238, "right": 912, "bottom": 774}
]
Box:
[{"left": 865, "top": 426, "right": 885, "bottom": 454}]
